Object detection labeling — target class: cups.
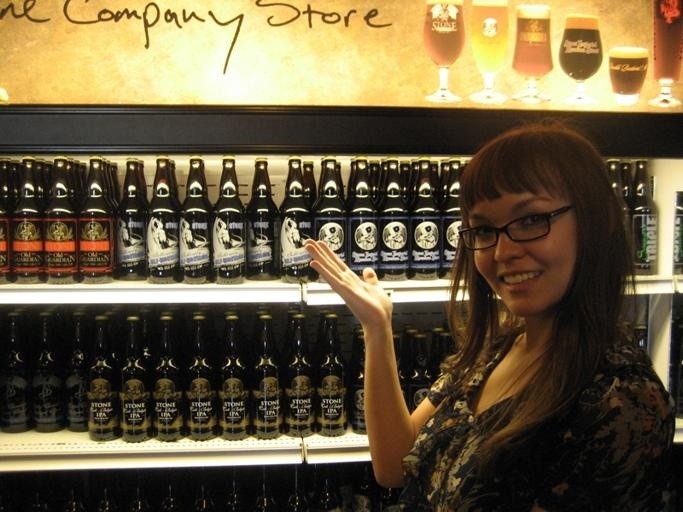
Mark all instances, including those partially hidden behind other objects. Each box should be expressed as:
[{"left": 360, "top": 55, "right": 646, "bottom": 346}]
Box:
[{"left": 608, "top": 47, "right": 648, "bottom": 106}]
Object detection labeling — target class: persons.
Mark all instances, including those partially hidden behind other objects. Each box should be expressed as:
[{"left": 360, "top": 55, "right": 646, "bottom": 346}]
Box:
[{"left": 302, "top": 123, "right": 677, "bottom": 512}]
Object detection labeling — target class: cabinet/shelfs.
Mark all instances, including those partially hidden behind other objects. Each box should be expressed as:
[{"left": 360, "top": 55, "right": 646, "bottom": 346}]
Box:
[{"left": 0, "top": 103, "right": 682, "bottom": 512}]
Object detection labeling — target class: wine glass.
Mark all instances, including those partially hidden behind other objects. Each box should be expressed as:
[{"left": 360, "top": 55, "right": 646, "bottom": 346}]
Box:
[
  {"left": 470, "top": 0, "right": 513, "bottom": 105},
  {"left": 558, "top": 15, "right": 604, "bottom": 104},
  {"left": 513, "top": 5, "right": 554, "bottom": 103},
  {"left": 422, "top": 0, "right": 466, "bottom": 102}
]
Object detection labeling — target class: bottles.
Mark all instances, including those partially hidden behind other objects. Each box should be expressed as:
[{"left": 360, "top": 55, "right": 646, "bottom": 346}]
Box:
[
  {"left": 672, "top": 471, "right": 683, "bottom": 512},
  {"left": 625, "top": 321, "right": 647, "bottom": 352},
  {"left": 670, "top": 315, "right": 683, "bottom": 418},
  {"left": 606, "top": 158, "right": 657, "bottom": 275},
  {"left": 673, "top": 191, "right": 683, "bottom": 275},
  {"left": 2, "top": 305, "right": 454, "bottom": 438},
  {"left": 0, "top": 158, "right": 466, "bottom": 289}
]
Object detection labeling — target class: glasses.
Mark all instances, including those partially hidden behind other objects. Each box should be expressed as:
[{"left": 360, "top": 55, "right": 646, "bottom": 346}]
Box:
[{"left": 460, "top": 204, "right": 573, "bottom": 251}]
